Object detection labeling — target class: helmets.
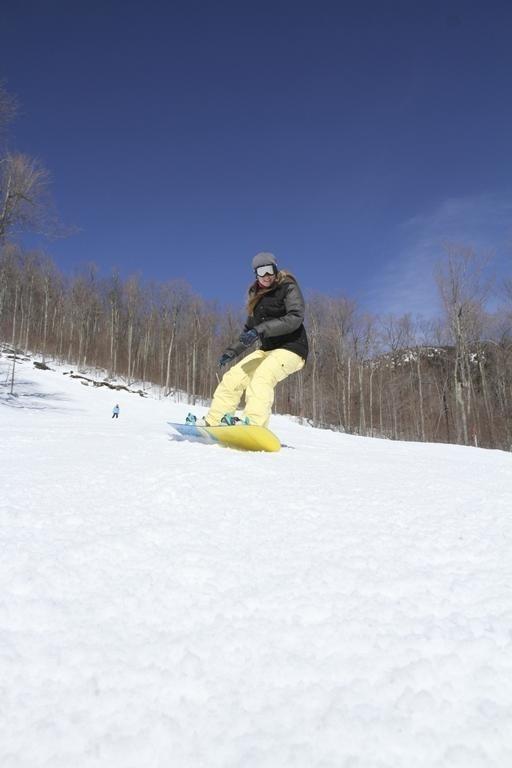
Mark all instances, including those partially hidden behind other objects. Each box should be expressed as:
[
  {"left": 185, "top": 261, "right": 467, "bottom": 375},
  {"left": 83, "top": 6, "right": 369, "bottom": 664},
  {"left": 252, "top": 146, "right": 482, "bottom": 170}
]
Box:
[{"left": 252, "top": 252, "right": 276, "bottom": 268}]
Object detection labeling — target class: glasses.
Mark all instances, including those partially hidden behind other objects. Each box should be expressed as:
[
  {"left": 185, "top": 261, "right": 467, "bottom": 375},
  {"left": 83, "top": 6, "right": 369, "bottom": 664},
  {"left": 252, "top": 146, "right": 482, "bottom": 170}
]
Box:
[{"left": 256, "top": 264, "right": 275, "bottom": 276}]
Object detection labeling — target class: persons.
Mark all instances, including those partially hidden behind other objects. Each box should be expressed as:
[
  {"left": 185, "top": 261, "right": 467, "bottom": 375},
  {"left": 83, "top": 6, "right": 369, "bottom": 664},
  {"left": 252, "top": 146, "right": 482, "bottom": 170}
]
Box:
[
  {"left": 184, "top": 410, "right": 243, "bottom": 426},
  {"left": 193, "top": 253, "right": 309, "bottom": 426},
  {"left": 111, "top": 403, "right": 120, "bottom": 418}
]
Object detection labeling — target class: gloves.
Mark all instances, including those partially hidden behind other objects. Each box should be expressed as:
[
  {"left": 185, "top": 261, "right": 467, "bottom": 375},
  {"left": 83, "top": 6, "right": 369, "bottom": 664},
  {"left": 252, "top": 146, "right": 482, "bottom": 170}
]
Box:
[
  {"left": 240, "top": 329, "right": 260, "bottom": 348},
  {"left": 218, "top": 352, "right": 235, "bottom": 369}
]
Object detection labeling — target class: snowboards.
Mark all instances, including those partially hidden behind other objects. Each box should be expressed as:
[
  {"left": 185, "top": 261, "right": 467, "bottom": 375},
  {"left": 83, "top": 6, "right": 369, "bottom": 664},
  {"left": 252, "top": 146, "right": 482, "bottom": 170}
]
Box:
[{"left": 166, "top": 423, "right": 281, "bottom": 452}]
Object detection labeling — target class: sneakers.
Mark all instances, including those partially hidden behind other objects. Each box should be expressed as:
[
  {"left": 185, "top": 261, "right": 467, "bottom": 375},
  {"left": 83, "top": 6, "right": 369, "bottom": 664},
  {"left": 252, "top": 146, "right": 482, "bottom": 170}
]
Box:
[
  {"left": 230, "top": 416, "right": 244, "bottom": 426},
  {"left": 195, "top": 418, "right": 209, "bottom": 426}
]
